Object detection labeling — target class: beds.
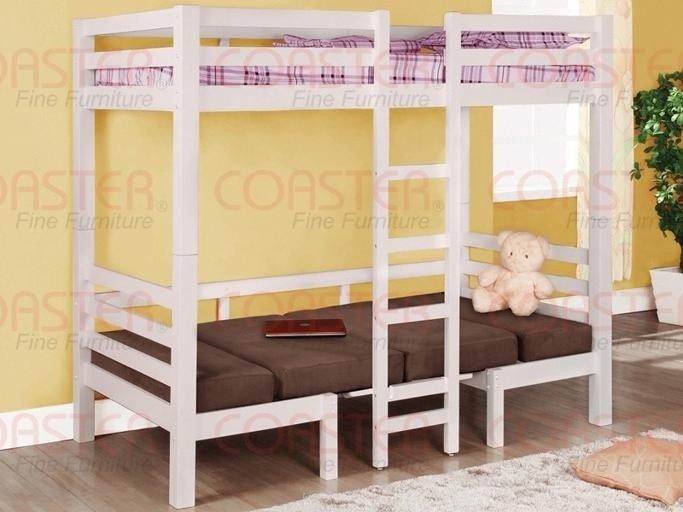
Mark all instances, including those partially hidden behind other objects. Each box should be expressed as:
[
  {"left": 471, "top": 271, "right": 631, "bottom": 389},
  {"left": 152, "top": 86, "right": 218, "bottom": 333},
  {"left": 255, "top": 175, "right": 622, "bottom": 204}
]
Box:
[{"left": 72, "top": 6, "right": 614, "bottom": 511}]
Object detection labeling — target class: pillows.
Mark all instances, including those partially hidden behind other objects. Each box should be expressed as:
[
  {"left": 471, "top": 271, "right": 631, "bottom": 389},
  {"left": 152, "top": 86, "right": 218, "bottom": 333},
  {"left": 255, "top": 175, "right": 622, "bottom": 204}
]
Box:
[{"left": 569, "top": 436, "right": 682, "bottom": 508}]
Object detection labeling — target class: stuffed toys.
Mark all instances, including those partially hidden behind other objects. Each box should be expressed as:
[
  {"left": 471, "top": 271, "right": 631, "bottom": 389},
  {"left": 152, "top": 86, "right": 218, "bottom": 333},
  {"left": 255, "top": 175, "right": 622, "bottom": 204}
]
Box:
[{"left": 472, "top": 229, "right": 554, "bottom": 316}]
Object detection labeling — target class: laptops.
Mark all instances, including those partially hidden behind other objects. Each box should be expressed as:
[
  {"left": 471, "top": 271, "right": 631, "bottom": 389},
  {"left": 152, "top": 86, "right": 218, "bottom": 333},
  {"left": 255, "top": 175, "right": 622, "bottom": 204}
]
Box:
[{"left": 264, "top": 318, "right": 348, "bottom": 340}]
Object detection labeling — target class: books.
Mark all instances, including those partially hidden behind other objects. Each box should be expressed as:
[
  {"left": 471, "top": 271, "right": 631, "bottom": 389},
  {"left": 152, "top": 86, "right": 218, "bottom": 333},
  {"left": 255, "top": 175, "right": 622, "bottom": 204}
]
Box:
[{"left": 264, "top": 319, "right": 347, "bottom": 337}]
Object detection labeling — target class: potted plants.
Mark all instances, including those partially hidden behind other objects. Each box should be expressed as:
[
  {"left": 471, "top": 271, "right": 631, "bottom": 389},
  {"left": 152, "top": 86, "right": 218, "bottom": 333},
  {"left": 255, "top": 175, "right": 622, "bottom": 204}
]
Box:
[{"left": 627, "top": 71, "right": 683, "bottom": 327}]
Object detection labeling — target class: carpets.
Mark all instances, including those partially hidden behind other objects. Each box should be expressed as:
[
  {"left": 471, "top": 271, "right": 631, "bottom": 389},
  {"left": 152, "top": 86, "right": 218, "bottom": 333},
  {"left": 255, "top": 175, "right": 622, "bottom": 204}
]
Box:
[{"left": 246, "top": 428, "right": 682, "bottom": 510}]
